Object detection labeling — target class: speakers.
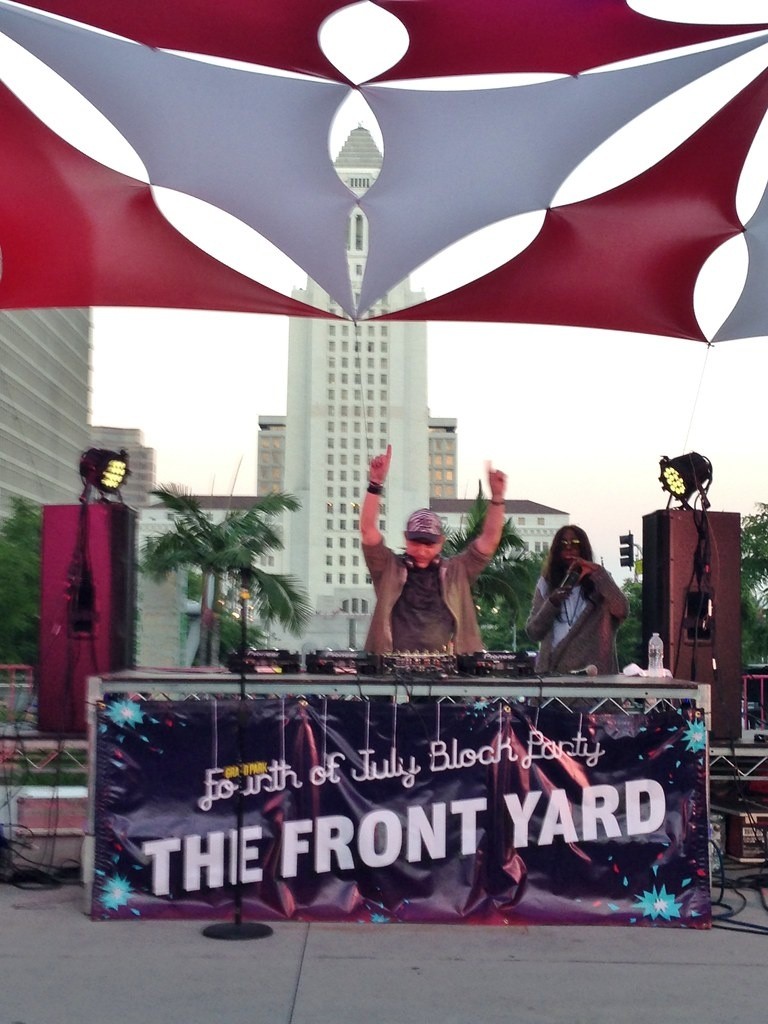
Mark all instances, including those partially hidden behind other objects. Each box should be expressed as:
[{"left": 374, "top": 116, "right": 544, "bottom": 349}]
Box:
[
  {"left": 639, "top": 509, "right": 743, "bottom": 739},
  {"left": 37, "top": 502, "right": 138, "bottom": 734}
]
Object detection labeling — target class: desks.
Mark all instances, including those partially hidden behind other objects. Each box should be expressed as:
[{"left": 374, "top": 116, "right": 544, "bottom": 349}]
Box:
[
  {"left": 708, "top": 739, "right": 768, "bottom": 781},
  {"left": 83, "top": 667, "right": 714, "bottom": 928}
]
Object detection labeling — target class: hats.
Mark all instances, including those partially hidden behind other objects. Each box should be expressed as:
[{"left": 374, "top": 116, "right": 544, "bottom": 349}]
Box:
[{"left": 403, "top": 508, "right": 444, "bottom": 542}]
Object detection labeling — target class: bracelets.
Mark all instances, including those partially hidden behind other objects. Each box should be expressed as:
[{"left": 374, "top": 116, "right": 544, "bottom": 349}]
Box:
[
  {"left": 490, "top": 500, "right": 505, "bottom": 506},
  {"left": 367, "top": 480, "right": 384, "bottom": 494}
]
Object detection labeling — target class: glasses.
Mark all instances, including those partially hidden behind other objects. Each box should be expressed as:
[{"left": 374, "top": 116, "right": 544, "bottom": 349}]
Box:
[{"left": 557, "top": 538, "right": 582, "bottom": 551}]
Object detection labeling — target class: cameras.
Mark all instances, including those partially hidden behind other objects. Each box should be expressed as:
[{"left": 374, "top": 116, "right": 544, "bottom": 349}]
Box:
[{"left": 559, "top": 570, "right": 578, "bottom": 589}]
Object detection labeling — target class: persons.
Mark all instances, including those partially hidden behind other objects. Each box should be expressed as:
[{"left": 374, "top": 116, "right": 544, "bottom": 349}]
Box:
[
  {"left": 526, "top": 525, "right": 630, "bottom": 678},
  {"left": 361, "top": 444, "right": 507, "bottom": 654}
]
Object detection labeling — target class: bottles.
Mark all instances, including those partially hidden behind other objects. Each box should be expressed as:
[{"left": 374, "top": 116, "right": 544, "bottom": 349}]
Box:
[{"left": 648, "top": 632, "right": 664, "bottom": 678}]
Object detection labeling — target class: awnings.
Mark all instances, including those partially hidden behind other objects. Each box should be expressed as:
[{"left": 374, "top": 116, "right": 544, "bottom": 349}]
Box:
[{"left": 0, "top": 1, "right": 766, "bottom": 345}]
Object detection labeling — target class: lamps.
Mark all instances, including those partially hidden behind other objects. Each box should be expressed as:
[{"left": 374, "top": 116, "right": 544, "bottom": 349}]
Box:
[
  {"left": 658, "top": 450, "right": 718, "bottom": 639},
  {"left": 65, "top": 445, "right": 132, "bottom": 635}
]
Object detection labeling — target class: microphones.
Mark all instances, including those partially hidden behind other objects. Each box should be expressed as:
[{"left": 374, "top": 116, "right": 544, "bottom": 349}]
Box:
[{"left": 240, "top": 571, "right": 253, "bottom": 603}]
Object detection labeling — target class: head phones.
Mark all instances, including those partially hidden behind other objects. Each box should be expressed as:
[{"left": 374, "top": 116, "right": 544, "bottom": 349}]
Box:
[{"left": 402, "top": 551, "right": 442, "bottom": 571}]
